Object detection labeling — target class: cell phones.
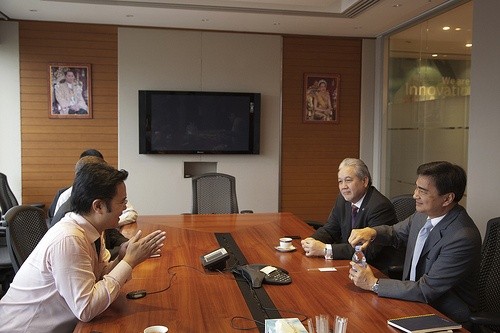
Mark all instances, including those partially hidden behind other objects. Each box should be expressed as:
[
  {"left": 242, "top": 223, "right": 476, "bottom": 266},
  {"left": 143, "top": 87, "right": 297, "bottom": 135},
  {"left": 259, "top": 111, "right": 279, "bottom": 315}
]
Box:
[{"left": 285, "top": 236, "right": 301, "bottom": 239}]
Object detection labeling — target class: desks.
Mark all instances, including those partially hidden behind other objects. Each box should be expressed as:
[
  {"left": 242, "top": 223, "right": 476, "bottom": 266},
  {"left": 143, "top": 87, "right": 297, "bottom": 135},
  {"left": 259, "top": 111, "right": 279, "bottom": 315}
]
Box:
[{"left": 73, "top": 210, "right": 466, "bottom": 333}]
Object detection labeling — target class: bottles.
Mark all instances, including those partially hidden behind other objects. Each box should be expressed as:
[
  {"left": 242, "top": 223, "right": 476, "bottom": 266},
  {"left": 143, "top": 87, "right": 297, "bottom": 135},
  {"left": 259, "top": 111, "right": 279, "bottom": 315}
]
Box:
[{"left": 351, "top": 246, "right": 366, "bottom": 272}]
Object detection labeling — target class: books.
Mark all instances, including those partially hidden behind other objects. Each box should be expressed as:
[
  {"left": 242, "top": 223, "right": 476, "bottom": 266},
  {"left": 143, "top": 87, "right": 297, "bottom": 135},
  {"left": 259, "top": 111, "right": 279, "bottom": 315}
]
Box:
[{"left": 387, "top": 313, "right": 462, "bottom": 333}]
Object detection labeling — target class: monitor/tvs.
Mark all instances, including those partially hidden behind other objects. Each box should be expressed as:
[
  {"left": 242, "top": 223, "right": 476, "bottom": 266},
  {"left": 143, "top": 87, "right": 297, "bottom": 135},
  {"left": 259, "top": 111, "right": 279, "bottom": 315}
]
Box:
[{"left": 138, "top": 90, "right": 260, "bottom": 155}]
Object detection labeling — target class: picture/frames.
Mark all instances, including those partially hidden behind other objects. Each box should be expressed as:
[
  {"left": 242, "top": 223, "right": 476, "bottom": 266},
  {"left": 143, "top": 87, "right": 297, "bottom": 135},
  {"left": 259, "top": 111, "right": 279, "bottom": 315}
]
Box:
[
  {"left": 48, "top": 63, "right": 93, "bottom": 118},
  {"left": 302, "top": 72, "right": 340, "bottom": 124}
]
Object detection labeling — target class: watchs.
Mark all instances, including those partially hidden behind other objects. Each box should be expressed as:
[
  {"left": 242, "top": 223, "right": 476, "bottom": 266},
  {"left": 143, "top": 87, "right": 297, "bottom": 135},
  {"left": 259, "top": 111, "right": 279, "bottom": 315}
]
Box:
[{"left": 372, "top": 278, "right": 380, "bottom": 294}]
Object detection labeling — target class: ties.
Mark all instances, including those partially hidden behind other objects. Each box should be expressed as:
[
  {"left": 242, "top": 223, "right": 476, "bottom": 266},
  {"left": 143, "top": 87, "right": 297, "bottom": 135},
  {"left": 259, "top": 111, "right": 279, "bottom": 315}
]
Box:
[
  {"left": 95, "top": 237, "right": 101, "bottom": 263},
  {"left": 409, "top": 220, "right": 433, "bottom": 282},
  {"left": 351, "top": 205, "right": 358, "bottom": 229}
]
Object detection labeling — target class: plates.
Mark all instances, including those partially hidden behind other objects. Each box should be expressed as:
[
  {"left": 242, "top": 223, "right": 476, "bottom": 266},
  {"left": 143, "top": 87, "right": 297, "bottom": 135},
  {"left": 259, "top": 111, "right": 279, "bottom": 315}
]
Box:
[{"left": 274, "top": 246, "right": 296, "bottom": 252}]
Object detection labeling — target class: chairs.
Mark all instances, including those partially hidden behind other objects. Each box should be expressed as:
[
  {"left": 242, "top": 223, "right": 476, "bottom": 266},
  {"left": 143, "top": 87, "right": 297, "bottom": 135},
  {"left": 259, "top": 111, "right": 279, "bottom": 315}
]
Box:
[
  {"left": 388, "top": 218, "right": 500, "bottom": 333},
  {"left": 392, "top": 194, "right": 416, "bottom": 220},
  {"left": 182, "top": 173, "right": 253, "bottom": 214},
  {"left": 0, "top": 173, "right": 49, "bottom": 296}
]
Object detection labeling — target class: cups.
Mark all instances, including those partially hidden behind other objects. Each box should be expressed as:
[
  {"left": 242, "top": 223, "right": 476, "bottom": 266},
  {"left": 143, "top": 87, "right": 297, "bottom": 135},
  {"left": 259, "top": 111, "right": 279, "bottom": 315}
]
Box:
[
  {"left": 279, "top": 237, "right": 292, "bottom": 248},
  {"left": 144, "top": 325, "right": 169, "bottom": 333}
]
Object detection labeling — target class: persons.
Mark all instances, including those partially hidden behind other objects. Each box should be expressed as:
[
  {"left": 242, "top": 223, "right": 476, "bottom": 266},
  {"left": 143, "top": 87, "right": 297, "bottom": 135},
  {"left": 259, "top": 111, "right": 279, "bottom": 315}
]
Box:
[
  {"left": 300, "top": 157, "right": 400, "bottom": 261},
  {"left": 347, "top": 161, "right": 483, "bottom": 323},
  {"left": 54, "top": 69, "right": 89, "bottom": 114},
  {"left": 44, "top": 148, "right": 137, "bottom": 262},
  {"left": 312, "top": 79, "right": 333, "bottom": 121},
  {"left": 0, "top": 164, "right": 167, "bottom": 332}
]
